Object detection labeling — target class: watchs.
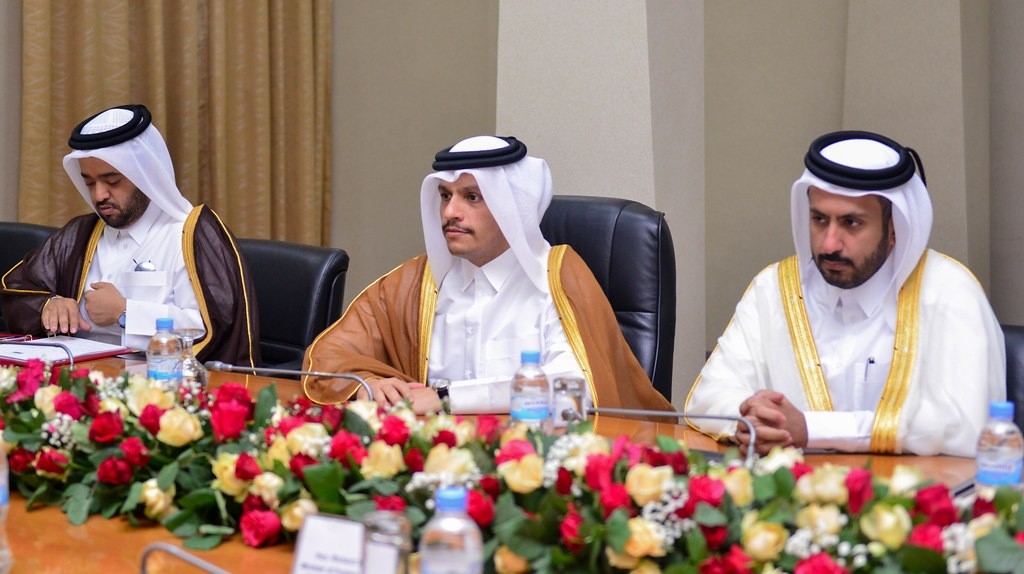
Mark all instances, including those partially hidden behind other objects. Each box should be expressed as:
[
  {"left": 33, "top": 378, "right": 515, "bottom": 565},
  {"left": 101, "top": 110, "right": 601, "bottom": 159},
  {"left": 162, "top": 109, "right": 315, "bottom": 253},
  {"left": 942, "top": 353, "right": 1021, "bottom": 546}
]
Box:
[
  {"left": 432, "top": 377, "right": 452, "bottom": 414},
  {"left": 117, "top": 311, "right": 126, "bottom": 329}
]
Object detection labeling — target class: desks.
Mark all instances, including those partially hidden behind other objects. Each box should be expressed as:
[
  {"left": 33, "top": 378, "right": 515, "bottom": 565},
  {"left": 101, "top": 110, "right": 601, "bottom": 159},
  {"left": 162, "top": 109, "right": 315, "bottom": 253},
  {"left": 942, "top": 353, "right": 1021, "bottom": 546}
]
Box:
[{"left": 0, "top": 356, "right": 978, "bottom": 574}]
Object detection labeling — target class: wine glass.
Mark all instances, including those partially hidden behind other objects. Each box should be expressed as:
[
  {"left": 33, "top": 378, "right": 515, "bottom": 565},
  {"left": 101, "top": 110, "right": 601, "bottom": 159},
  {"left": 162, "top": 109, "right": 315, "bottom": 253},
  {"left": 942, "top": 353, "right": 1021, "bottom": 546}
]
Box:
[{"left": 174, "top": 330, "right": 207, "bottom": 393}]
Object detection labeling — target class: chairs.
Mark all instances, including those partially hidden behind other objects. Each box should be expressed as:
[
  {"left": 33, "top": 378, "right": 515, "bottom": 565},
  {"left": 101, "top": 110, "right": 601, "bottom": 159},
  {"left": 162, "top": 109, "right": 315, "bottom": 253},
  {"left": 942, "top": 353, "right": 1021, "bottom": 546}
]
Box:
[
  {"left": 540, "top": 195, "right": 677, "bottom": 403},
  {"left": 0, "top": 221, "right": 61, "bottom": 282},
  {"left": 234, "top": 238, "right": 349, "bottom": 379}
]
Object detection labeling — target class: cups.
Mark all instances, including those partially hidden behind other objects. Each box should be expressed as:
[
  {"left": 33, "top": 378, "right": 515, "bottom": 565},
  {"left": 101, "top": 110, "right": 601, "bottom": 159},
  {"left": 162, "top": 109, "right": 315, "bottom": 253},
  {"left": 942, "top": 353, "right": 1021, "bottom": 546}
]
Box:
[
  {"left": 551, "top": 377, "right": 586, "bottom": 434},
  {"left": 360, "top": 511, "right": 412, "bottom": 574}
]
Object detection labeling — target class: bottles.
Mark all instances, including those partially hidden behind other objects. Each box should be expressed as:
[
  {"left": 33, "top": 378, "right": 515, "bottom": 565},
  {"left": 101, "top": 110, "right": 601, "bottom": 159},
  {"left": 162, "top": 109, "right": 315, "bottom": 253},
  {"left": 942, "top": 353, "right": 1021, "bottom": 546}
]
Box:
[
  {"left": 420, "top": 485, "right": 483, "bottom": 574},
  {"left": 972, "top": 401, "right": 1024, "bottom": 505},
  {"left": 508, "top": 350, "right": 550, "bottom": 435},
  {"left": 0, "top": 432, "right": 12, "bottom": 574},
  {"left": 146, "top": 317, "right": 183, "bottom": 391}
]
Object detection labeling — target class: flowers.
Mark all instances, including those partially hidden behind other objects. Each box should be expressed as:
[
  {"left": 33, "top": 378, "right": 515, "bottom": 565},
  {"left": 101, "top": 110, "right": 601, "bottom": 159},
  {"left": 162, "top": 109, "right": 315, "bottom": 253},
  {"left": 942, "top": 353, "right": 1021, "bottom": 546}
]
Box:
[{"left": 0, "top": 357, "right": 1024, "bottom": 574}]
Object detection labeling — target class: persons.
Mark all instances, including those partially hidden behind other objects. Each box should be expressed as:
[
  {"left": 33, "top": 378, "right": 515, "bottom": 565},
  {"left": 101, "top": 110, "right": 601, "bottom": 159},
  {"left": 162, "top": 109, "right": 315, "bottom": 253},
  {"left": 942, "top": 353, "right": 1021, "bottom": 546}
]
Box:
[
  {"left": 1, "top": 103, "right": 259, "bottom": 376},
  {"left": 300, "top": 134, "right": 678, "bottom": 424},
  {"left": 683, "top": 129, "right": 1007, "bottom": 458}
]
